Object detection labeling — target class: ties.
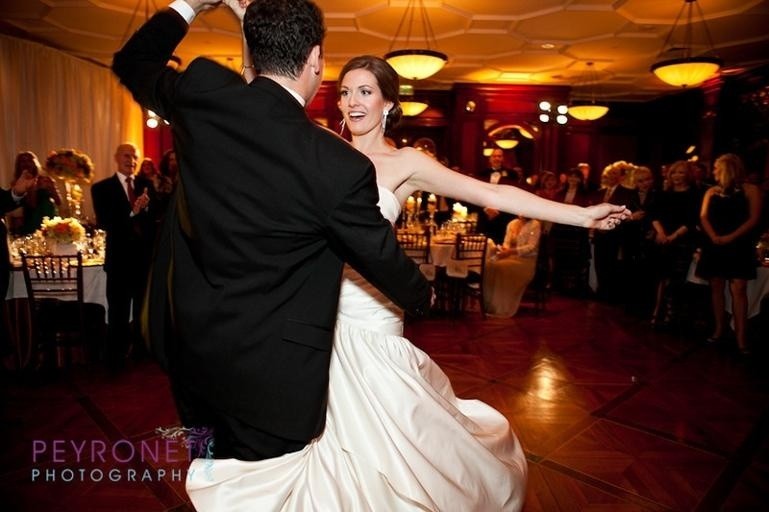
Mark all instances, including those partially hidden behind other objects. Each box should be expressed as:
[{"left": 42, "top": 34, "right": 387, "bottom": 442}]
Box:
[{"left": 125, "top": 177, "right": 135, "bottom": 209}]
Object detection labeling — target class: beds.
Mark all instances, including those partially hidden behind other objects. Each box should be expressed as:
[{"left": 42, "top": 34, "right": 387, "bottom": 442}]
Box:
[{"left": 724, "top": 257, "right": 769, "bottom": 331}]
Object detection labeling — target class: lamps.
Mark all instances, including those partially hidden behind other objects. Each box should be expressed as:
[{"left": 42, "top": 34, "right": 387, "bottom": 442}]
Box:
[
  {"left": 395, "top": 85, "right": 430, "bottom": 118},
  {"left": 118, "top": 1, "right": 183, "bottom": 74},
  {"left": 145, "top": 109, "right": 171, "bottom": 129},
  {"left": 567, "top": 62, "right": 611, "bottom": 122},
  {"left": 383, "top": 1, "right": 448, "bottom": 83},
  {"left": 647, "top": 0, "right": 722, "bottom": 89}
]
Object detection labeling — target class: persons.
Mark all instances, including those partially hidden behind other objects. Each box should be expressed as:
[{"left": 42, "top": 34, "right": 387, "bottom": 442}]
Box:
[
  {"left": 109, "top": 0, "right": 441, "bottom": 459},
  {"left": 218, "top": 0, "right": 635, "bottom": 512},
  {"left": 0, "top": 142, "right": 768, "bottom": 369}
]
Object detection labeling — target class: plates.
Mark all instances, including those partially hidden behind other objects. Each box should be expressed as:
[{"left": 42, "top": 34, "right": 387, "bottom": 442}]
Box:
[{"left": 71, "top": 261, "right": 104, "bottom": 266}]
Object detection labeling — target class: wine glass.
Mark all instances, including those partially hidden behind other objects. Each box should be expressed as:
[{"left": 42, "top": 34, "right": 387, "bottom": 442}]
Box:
[
  {"left": 9, "top": 229, "right": 105, "bottom": 262},
  {"left": 439, "top": 220, "right": 466, "bottom": 240}
]
